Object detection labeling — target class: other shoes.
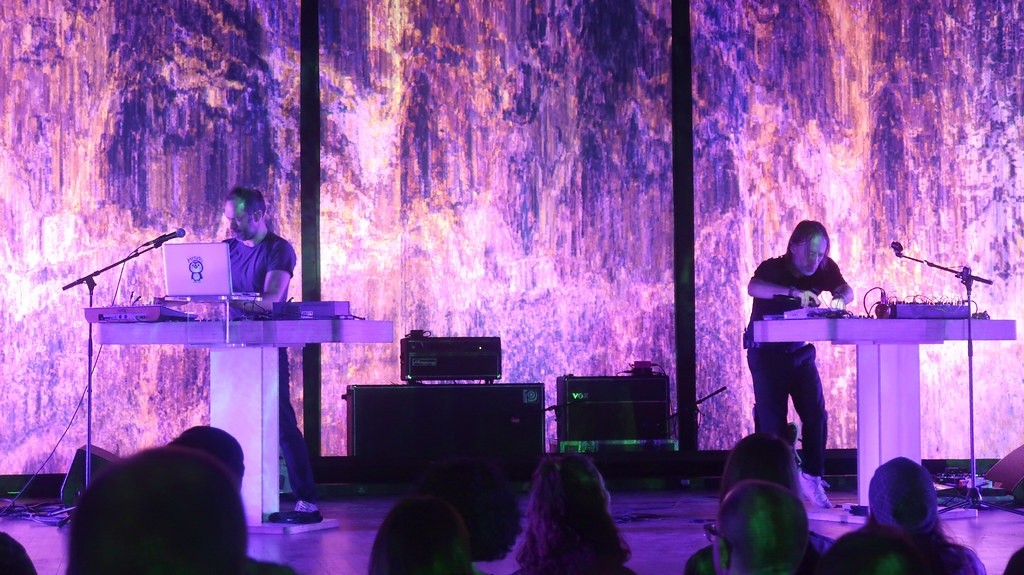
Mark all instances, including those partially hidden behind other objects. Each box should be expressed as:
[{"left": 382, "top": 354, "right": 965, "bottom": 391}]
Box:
[{"left": 295, "top": 501, "right": 319, "bottom": 516}]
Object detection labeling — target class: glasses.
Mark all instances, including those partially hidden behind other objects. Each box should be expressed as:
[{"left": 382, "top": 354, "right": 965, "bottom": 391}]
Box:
[{"left": 702, "top": 522, "right": 735, "bottom": 552}]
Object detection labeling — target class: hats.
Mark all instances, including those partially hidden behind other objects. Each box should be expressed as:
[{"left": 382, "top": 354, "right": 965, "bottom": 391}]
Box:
[{"left": 869, "top": 453, "right": 938, "bottom": 534}]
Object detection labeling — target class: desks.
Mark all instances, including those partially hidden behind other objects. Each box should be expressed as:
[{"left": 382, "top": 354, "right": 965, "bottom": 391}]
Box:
[
  {"left": 753, "top": 320, "right": 1016, "bottom": 499},
  {"left": 97, "top": 321, "right": 393, "bottom": 534}
]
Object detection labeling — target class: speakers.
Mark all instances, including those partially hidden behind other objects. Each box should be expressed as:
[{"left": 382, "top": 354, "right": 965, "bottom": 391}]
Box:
[
  {"left": 400, "top": 337, "right": 502, "bottom": 380},
  {"left": 986, "top": 444, "right": 1024, "bottom": 507},
  {"left": 554, "top": 375, "right": 670, "bottom": 444},
  {"left": 345, "top": 382, "right": 546, "bottom": 460}
]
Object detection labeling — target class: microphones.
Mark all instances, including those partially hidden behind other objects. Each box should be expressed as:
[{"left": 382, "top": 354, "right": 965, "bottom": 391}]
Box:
[
  {"left": 142, "top": 228, "right": 186, "bottom": 246},
  {"left": 773, "top": 294, "right": 820, "bottom": 307}
]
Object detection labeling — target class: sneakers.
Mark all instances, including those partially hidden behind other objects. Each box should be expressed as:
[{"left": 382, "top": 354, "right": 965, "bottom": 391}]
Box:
[{"left": 796, "top": 467, "right": 831, "bottom": 509}]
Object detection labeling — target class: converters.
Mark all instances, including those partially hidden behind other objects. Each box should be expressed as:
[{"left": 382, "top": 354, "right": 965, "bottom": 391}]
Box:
[{"left": 631, "top": 361, "right": 653, "bottom": 376}]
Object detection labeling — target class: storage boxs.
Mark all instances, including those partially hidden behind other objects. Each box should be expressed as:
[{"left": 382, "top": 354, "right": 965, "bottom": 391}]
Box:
[
  {"left": 557, "top": 377, "right": 669, "bottom": 440},
  {"left": 347, "top": 383, "right": 545, "bottom": 484}
]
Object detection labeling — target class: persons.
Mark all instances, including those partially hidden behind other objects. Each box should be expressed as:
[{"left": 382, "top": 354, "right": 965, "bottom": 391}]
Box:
[
  {"left": 218, "top": 188, "right": 319, "bottom": 512},
  {"left": 0, "top": 421, "right": 1024, "bottom": 575},
  {"left": 742, "top": 220, "right": 854, "bottom": 505}
]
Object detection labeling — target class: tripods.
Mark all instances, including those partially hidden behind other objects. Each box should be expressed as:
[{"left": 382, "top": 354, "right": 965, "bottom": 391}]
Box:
[{"left": 890, "top": 241, "right": 1024, "bottom": 516}]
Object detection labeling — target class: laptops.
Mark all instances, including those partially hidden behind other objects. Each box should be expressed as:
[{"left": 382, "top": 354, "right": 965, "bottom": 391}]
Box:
[{"left": 162, "top": 242, "right": 260, "bottom": 296}]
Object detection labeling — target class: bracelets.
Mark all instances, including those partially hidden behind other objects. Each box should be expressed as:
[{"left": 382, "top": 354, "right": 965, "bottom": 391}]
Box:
[{"left": 789, "top": 287, "right": 797, "bottom": 300}]
